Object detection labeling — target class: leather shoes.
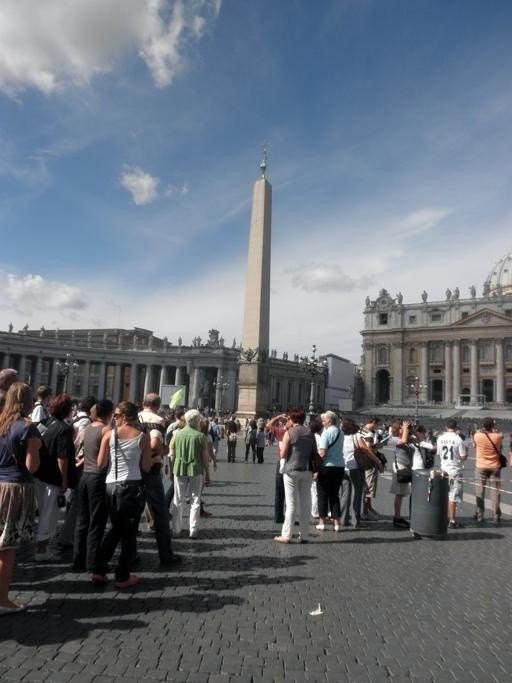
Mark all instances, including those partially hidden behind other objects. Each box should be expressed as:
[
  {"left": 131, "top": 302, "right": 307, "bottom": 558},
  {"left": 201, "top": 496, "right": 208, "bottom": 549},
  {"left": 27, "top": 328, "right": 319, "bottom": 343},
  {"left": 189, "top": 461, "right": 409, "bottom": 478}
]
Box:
[
  {"left": 0, "top": 603, "right": 27, "bottom": 614},
  {"left": 274, "top": 535, "right": 291, "bottom": 542},
  {"left": 333, "top": 524, "right": 340, "bottom": 531},
  {"left": 316, "top": 524, "right": 325, "bottom": 530}
]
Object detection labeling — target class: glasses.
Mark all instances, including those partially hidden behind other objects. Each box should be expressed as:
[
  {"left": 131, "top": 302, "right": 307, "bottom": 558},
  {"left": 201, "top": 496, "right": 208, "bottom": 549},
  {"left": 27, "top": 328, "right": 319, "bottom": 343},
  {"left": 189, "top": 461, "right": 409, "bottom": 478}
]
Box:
[{"left": 114, "top": 413, "right": 122, "bottom": 416}]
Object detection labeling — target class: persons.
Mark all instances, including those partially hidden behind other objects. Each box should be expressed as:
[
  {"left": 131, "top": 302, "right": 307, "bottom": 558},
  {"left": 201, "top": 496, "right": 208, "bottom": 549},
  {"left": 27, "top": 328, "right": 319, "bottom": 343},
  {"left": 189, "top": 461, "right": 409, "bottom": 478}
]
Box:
[
  {"left": 2, "top": 368, "right": 229, "bottom": 610},
  {"left": 437, "top": 419, "right": 468, "bottom": 528},
  {"left": 365, "top": 284, "right": 498, "bottom": 307},
  {"left": 266, "top": 410, "right": 437, "bottom": 545},
  {"left": 8, "top": 324, "right": 328, "bottom": 367},
  {"left": 471, "top": 416, "right": 503, "bottom": 523},
  {"left": 224, "top": 415, "right": 265, "bottom": 464}
]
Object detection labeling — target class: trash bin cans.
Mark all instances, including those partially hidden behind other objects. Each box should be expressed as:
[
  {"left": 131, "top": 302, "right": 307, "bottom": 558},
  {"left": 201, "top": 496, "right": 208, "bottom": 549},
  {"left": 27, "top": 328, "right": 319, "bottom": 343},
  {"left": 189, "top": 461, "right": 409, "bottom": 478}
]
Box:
[{"left": 410, "top": 469, "right": 449, "bottom": 540}]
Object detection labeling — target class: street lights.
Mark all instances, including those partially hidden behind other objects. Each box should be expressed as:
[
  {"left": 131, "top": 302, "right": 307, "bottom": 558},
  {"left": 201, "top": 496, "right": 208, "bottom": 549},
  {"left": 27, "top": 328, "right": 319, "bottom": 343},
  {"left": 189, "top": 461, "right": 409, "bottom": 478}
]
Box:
[
  {"left": 406, "top": 376, "right": 428, "bottom": 425},
  {"left": 212, "top": 374, "right": 230, "bottom": 413},
  {"left": 55, "top": 352, "right": 80, "bottom": 395},
  {"left": 298, "top": 344, "right": 329, "bottom": 414}
]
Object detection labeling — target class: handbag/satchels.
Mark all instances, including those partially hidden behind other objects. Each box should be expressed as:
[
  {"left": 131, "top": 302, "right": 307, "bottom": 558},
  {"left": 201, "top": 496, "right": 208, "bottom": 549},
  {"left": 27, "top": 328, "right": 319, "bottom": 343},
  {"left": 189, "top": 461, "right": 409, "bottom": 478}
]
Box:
[
  {"left": 499, "top": 453, "right": 507, "bottom": 466},
  {"left": 375, "top": 451, "right": 387, "bottom": 469},
  {"left": 353, "top": 432, "right": 374, "bottom": 470},
  {"left": 307, "top": 451, "right": 324, "bottom": 472},
  {"left": 113, "top": 479, "right": 146, "bottom": 515},
  {"left": 397, "top": 468, "right": 414, "bottom": 482}
]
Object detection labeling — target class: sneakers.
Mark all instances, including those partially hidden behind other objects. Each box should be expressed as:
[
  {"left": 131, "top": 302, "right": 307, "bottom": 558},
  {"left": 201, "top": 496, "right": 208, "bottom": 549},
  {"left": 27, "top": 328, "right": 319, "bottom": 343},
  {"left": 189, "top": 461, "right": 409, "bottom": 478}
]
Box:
[
  {"left": 393, "top": 519, "right": 410, "bottom": 527},
  {"left": 114, "top": 574, "right": 138, "bottom": 587},
  {"left": 361, "top": 510, "right": 379, "bottom": 520},
  {"left": 448, "top": 520, "right": 465, "bottom": 528},
  {"left": 394, "top": 516, "right": 410, "bottom": 524},
  {"left": 88, "top": 572, "right": 108, "bottom": 582}
]
